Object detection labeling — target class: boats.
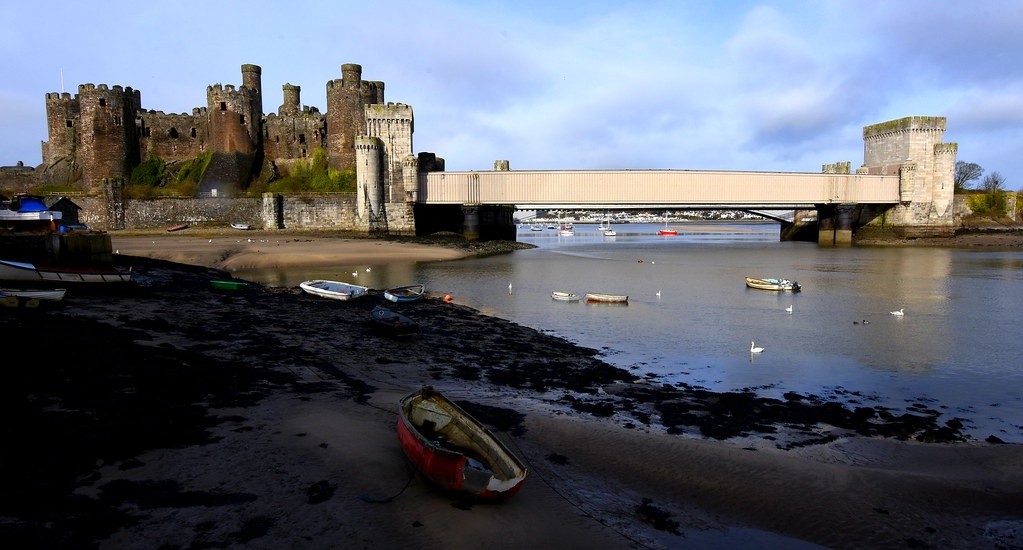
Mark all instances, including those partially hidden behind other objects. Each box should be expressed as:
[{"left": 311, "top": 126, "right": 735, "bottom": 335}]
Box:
[
  {"left": 210, "top": 276, "right": 251, "bottom": 293},
  {"left": 394, "top": 384, "right": 528, "bottom": 507},
  {"left": 658, "top": 212, "right": 679, "bottom": 235},
  {"left": 0, "top": 192, "right": 63, "bottom": 232},
  {"left": 300, "top": 279, "right": 369, "bottom": 302},
  {"left": 383, "top": 283, "right": 426, "bottom": 303},
  {"left": 598, "top": 209, "right": 617, "bottom": 236},
  {"left": 0, "top": 259, "right": 133, "bottom": 292},
  {"left": 230, "top": 223, "right": 252, "bottom": 230},
  {"left": 586, "top": 293, "right": 629, "bottom": 303},
  {"left": 0, "top": 284, "right": 69, "bottom": 310},
  {"left": 744, "top": 275, "right": 802, "bottom": 291},
  {"left": 167, "top": 223, "right": 188, "bottom": 231},
  {"left": 551, "top": 291, "right": 580, "bottom": 302},
  {"left": 529, "top": 210, "right": 575, "bottom": 234},
  {"left": 370, "top": 305, "right": 420, "bottom": 331}
]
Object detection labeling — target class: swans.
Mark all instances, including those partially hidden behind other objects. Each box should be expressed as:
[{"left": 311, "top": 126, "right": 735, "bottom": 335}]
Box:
[
  {"left": 656, "top": 290, "right": 662, "bottom": 296},
  {"left": 352, "top": 270, "right": 359, "bottom": 276},
  {"left": 890, "top": 308, "right": 905, "bottom": 316},
  {"left": 785, "top": 305, "right": 792, "bottom": 312},
  {"left": 365, "top": 267, "right": 372, "bottom": 272},
  {"left": 750, "top": 341, "right": 764, "bottom": 353}
]
